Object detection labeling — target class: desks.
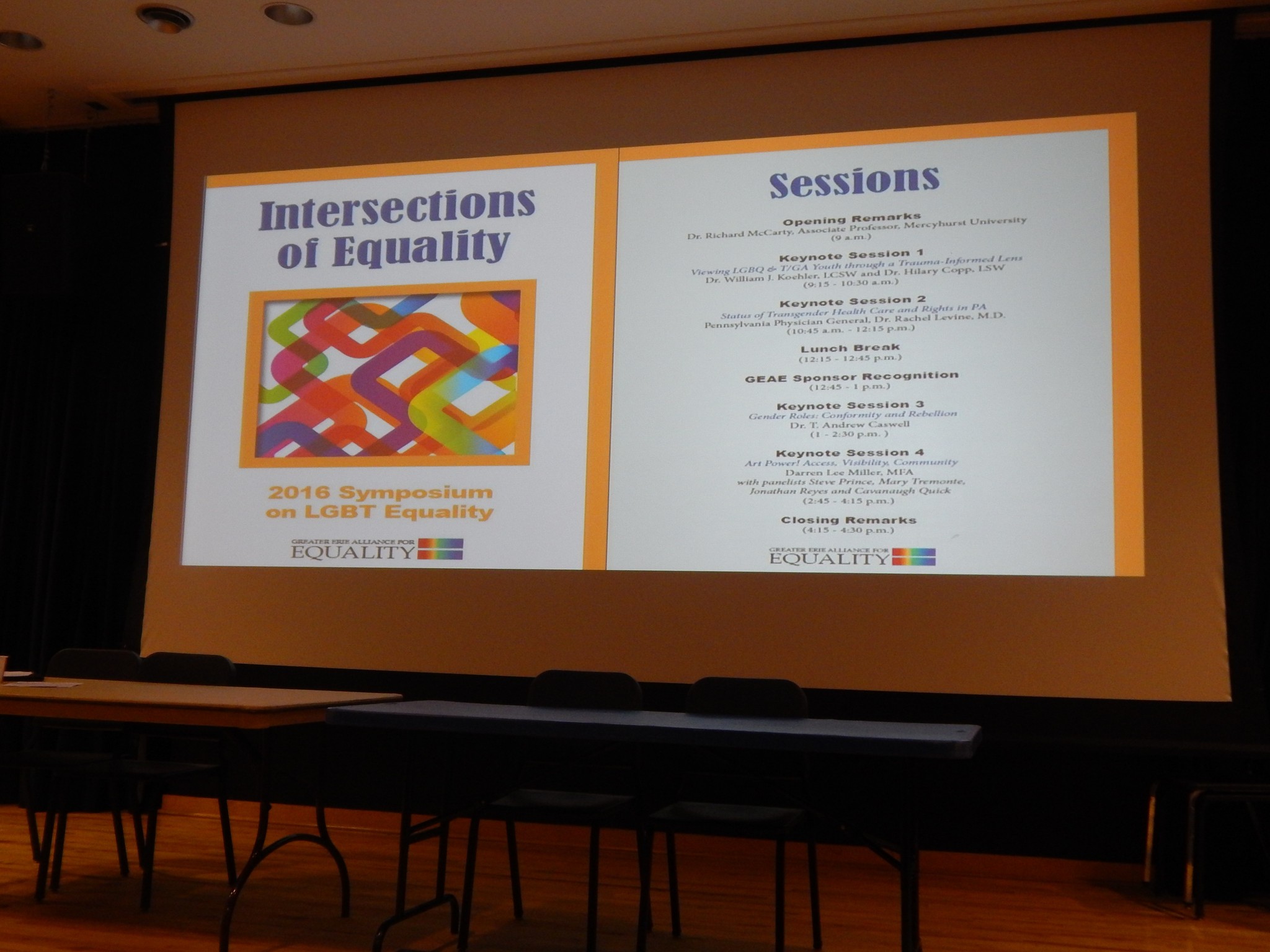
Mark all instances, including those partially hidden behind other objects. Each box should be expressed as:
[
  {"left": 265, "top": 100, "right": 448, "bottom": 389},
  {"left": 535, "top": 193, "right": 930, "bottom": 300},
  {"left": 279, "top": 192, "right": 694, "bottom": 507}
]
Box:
[
  {"left": 0, "top": 674, "right": 403, "bottom": 952},
  {"left": 321, "top": 700, "right": 984, "bottom": 952}
]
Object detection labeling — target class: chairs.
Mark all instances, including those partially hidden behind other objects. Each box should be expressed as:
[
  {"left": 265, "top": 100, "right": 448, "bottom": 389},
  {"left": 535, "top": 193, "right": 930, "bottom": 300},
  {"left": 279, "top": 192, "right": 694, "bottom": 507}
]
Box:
[
  {"left": 635, "top": 675, "right": 823, "bottom": 952},
  {"left": 455, "top": 669, "right": 653, "bottom": 952},
  {"left": 31, "top": 651, "right": 241, "bottom": 914},
  {"left": 0, "top": 649, "right": 147, "bottom": 890}
]
